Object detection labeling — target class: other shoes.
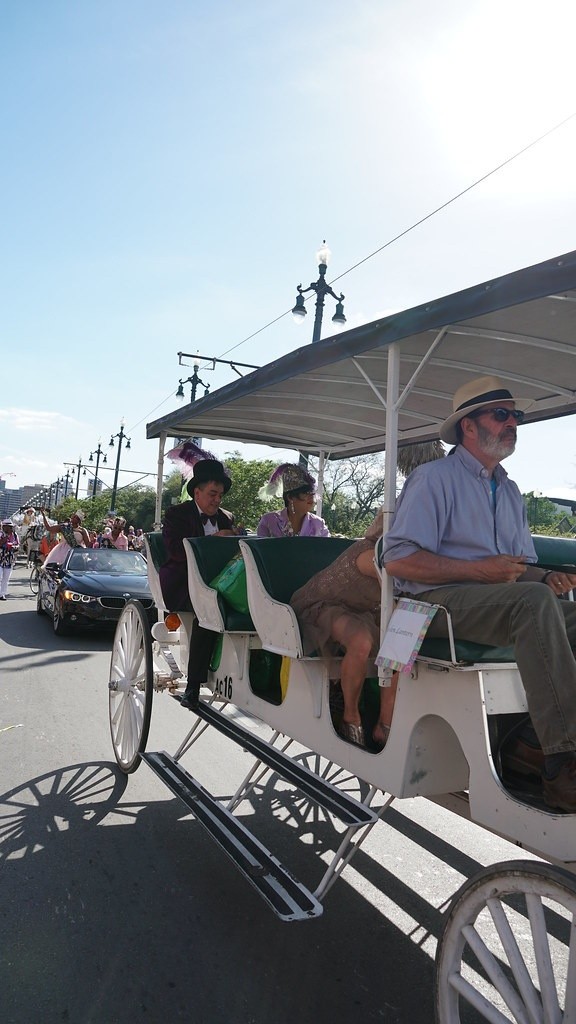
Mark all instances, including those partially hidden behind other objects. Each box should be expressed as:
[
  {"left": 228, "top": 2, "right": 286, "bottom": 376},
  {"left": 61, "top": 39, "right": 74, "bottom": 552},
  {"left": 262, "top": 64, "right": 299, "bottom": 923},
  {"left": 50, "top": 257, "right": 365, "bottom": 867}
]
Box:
[
  {"left": 0, "top": 595, "right": 6, "bottom": 600},
  {"left": 342, "top": 718, "right": 365, "bottom": 746},
  {"left": 372, "top": 721, "right": 391, "bottom": 747},
  {"left": 542, "top": 760, "right": 576, "bottom": 813}
]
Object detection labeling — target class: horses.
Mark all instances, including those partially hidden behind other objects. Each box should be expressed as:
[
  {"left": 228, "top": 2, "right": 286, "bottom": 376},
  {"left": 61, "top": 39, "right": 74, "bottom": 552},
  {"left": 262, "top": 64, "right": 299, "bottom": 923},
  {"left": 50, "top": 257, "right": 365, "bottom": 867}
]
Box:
[{"left": 26, "top": 523, "right": 46, "bottom": 569}]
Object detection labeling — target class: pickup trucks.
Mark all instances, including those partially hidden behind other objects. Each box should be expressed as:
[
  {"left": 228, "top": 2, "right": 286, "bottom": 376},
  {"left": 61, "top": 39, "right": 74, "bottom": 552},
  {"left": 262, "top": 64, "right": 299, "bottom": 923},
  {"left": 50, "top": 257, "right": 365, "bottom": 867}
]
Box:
[{"left": 35, "top": 542, "right": 160, "bottom": 637}]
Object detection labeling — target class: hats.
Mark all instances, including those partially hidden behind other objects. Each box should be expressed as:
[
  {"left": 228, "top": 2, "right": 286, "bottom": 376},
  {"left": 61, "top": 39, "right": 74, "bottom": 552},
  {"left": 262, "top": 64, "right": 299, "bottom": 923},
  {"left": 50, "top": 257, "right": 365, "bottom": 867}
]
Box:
[
  {"left": 24, "top": 507, "right": 37, "bottom": 513},
  {"left": 440, "top": 376, "right": 535, "bottom": 444},
  {"left": 167, "top": 442, "right": 232, "bottom": 499},
  {"left": 71, "top": 509, "right": 86, "bottom": 521},
  {"left": 258, "top": 463, "right": 316, "bottom": 501},
  {"left": 114, "top": 517, "right": 126, "bottom": 527},
  {"left": 2, "top": 519, "right": 15, "bottom": 526}
]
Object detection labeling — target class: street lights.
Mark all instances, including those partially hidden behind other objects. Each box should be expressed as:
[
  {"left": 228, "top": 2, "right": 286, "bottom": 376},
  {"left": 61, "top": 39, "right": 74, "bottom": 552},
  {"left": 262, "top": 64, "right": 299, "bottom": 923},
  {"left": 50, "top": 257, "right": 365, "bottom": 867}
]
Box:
[
  {"left": 72, "top": 456, "right": 87, "bottom": 500},
  {"left": 33, "top": 475, "right": 64, "bottom": 509},
  {"left": 172, "top": 349, "right": 211, "bottom": 498},
  {"left": 60, "top": 469, "right": 74, "bottom": 501},
  {"left": 88, "top": 436, "right": 108, "bottom": 505},
  {"left": 108, "top": 415, "right": 132, "bottom": 519},
  {"left": 288, "top": 237, "right": 346, "bottom": 474}
]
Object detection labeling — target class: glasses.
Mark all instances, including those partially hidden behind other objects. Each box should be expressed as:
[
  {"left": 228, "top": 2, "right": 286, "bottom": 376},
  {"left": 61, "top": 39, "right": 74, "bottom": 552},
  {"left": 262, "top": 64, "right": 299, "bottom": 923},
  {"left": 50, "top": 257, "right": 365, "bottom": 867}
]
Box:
[
  {"left": 468, "top": 407, "right": 524, "bottom": 421},
  {"left": 114, "top": 527, "right": 122, "bottom": 531}
]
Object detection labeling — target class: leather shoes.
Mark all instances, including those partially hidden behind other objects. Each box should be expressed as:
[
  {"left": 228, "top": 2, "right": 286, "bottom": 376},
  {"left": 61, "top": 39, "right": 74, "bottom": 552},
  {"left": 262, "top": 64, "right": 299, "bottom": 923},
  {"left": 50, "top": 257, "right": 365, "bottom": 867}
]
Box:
[{"left": 181, "top": 683, "right": 199, "bottom": 708}]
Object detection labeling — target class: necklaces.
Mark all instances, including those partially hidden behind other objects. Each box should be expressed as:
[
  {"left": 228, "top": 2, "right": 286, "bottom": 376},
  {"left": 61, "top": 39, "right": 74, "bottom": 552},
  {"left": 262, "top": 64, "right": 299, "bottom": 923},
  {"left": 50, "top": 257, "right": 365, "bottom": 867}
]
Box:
[{"left": 292, "top": 527, "right": 300, "bottom": 534}]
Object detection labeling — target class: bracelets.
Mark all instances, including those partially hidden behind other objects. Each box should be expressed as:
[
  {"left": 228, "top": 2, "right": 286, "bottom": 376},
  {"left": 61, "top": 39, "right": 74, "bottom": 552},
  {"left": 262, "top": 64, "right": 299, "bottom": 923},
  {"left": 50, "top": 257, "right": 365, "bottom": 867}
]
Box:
[{"left": 541, "top": 571, "right": 552, "bottom": 583}]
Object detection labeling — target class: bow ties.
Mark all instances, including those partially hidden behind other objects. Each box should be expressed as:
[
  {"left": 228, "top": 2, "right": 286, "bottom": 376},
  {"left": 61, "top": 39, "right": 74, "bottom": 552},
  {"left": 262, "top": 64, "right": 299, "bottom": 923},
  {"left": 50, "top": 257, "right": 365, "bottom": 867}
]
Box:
[{"left": 201, "top": 513, "right": 217, "bottom": 526}]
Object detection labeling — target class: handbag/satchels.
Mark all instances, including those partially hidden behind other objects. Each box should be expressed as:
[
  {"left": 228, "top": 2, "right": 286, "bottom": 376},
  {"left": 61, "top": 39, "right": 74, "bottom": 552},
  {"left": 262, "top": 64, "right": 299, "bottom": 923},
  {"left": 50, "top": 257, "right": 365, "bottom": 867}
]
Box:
[{"left": 209, "top": 550, "right": 249, "bottom": 614}]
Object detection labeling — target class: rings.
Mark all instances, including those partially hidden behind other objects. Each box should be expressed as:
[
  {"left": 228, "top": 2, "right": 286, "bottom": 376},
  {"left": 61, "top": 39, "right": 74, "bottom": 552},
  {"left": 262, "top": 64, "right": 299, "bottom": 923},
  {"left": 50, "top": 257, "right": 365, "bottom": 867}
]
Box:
[{"left": 555, "top": 582, "right": 561, "bottom": 584}]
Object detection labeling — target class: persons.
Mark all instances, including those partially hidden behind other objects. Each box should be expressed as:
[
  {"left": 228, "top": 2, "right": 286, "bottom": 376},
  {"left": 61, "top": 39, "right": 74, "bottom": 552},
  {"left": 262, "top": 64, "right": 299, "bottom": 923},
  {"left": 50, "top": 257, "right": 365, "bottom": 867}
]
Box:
[
  {"left": 289, "top": 494, "right": 400, "bottom": 747},
  {"left": 379, "top": 377, "right": 576, "bottom": 813},
  {"left": 158, "top": 458, "right": 249, "bottom": 710},
  {"left": 257, "top": 463, "right": 330, "bottom": 537},
  {"left": 0, "top": 507, "right": 144, "bottom": 600}
]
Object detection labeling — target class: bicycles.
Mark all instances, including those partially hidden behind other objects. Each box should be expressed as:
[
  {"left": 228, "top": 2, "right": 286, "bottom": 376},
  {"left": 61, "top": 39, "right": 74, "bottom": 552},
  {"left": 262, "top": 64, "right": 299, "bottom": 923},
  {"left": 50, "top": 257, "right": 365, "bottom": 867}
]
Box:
[{"left": 28, "top": 555, "right": 44, "bottom": 596}]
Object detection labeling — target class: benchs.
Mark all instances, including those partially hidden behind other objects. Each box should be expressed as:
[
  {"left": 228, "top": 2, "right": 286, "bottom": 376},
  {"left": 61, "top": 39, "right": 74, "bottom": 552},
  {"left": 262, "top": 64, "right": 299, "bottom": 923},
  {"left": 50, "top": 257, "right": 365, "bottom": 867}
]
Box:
[
  {"left": 144, "top": 532, "right": 209, "bottom": 676},
  {"left": 183, "top": 535, "right": 286, "bottom": 701},
  {"left": 373, "top": 536, "right": 575, "bottom": 715},
  {"left": 238, "top": 535, "right": 376, "bottom": 727}
]
